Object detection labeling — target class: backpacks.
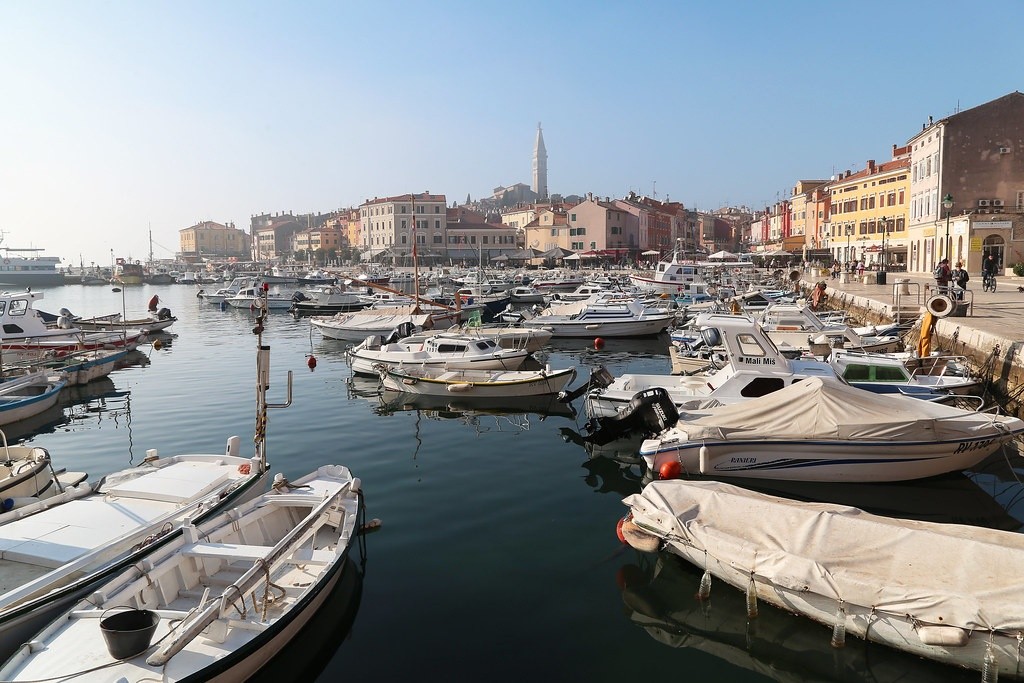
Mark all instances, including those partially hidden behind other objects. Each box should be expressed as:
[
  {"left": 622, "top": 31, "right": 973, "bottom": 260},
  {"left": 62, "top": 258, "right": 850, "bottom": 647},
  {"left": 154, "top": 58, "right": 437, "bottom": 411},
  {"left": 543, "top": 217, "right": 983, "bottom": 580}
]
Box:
[{"left": 934, "top": 265, "right": 947, "bottom": 278}]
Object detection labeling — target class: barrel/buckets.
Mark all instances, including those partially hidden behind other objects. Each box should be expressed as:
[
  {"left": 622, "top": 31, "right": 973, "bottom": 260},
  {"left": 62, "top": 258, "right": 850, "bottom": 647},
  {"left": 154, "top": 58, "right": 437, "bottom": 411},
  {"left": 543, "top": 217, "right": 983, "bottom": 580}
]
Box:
[{"left": 99, "top": 606, "right": 161, "bottom": 660}]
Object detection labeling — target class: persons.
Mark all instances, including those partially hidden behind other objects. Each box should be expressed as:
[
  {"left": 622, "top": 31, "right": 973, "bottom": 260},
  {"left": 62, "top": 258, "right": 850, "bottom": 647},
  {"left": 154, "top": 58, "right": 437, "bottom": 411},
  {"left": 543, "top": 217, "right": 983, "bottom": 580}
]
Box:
[
  {"left": 601, "top": 259, "right": 655, "bottom": 271},
  {"left": 832, "top": 259, "right": 865, "bottom": 279},
  {"left": 496, "top": 261, "right": 504, "bottom": 270},
  {"left": 787, "top": 259, "right": 793, "bottom": 272},
  {"left": 936, "top": 259, "right": 951, "bottom": 294},
  {"left": 951, "top": 262, "right": 969, "bottom": 300},
  {"left": 800, "top": 259, "right": 828, "bottom": 275},
  {"left": 764, "top": 258, "right": 777, "bottom": 271},
  {"left": 982, "top": 254, "right": 996, "bottom": 286}
]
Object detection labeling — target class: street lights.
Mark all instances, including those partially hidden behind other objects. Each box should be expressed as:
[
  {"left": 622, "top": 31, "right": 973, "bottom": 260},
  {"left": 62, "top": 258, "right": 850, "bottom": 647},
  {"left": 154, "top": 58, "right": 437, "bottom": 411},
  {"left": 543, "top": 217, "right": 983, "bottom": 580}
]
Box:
[
  {"left": 811, "top": 237, "right": 816, "bottom": 265},
  {"left": 941, "top": 194, "right": 956, "bottom": 259},
  {"left": 879, "top": 217, "right": 890, "bottom": 271},
  {"left": 825, "top": 232, "right": 831, "bottom": 267},
  {"left": 845, "top": 225, "right": 853, "bottom": 262}
]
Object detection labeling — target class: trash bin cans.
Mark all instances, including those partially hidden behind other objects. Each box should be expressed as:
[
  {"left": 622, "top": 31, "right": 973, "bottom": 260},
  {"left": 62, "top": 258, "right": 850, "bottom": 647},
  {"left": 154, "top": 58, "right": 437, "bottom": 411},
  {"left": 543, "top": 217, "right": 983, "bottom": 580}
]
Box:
[
  {"left": 952, "top": 288, "right": 963, "bottom": 301},
  {"left": 877, "top": 272, "right": 886, "bottom": 284}
]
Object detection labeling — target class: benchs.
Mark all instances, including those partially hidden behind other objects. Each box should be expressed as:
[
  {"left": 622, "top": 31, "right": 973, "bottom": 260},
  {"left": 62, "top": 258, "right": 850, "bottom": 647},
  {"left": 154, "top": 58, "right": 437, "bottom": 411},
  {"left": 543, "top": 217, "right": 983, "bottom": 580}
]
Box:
[
  {"left": 648, "top": 385, "right": 688, "bottom": 395},
  {"left": 435, "top": 371, "right": 460, "bottom": 380},
  {"left": 180, "top": 540, "right": 335, "bottom": 565}
]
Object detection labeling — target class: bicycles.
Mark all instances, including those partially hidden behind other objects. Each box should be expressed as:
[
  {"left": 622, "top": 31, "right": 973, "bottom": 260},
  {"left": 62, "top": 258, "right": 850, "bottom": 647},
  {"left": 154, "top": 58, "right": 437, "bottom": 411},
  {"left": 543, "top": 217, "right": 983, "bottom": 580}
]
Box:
[{"left": 981, "top": 271, "right": 996, "bottom": 293}]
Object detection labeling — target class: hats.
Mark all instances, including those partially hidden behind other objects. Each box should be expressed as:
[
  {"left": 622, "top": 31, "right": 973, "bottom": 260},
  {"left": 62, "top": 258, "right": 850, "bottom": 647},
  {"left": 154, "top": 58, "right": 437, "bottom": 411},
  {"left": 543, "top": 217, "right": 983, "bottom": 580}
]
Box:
[{"left": 955, "top": 262, "right": 962, "bottom": 266}]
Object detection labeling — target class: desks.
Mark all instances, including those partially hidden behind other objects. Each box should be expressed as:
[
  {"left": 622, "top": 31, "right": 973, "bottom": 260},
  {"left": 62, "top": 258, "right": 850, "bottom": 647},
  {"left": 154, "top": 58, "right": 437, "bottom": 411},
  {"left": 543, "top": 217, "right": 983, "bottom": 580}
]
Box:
[{"left": 680, "top": 376, "right": 706, "bottom": 395}]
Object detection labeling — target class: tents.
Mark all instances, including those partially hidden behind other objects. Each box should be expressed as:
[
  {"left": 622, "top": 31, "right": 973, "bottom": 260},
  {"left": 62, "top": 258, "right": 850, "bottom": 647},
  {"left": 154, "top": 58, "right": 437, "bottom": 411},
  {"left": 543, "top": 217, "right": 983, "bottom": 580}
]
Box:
[
  {"left": 490, "top": 245, "right": 660, "bottom": 265},
  {"left": 708, "top": 250, "right": 794, "bottom": 263}
]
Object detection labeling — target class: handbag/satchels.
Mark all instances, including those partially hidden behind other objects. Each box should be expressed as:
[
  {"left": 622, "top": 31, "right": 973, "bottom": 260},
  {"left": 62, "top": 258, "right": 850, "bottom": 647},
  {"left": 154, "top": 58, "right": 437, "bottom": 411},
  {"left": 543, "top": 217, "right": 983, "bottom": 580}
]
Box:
[
  {"left": 953, "top": 280, "right": 964, "bottom": 294},
  {"left": 861, "top": 262, "right": 864, "bottom": 267}
]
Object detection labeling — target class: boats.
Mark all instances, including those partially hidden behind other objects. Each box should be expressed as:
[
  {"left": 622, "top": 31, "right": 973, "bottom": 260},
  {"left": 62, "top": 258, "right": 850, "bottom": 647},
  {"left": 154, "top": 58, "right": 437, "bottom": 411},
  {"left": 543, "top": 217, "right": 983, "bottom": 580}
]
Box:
[
  {"left": 617, "top": 478, "right": 1024, "bottom": 679},
  {"left": 344, "top": 371, "right": 382, "bottom": 402},
  {"left": 79, "top": 241, "right": 1024, "bottom": 483},
  {"left": 1, "top": 435, "right": 271, "bottom": 665},
  {"left": 618, "top": 550, "right": 1022, "bottom": 683},
  {"left": 1, "top": 286, "right": 177, "bottom": 426},
  {"left": 1, "top": 465, "right": 364, "bottom": 683},
  {"left": 0, "top": 441, "right": 87, "bottom": 514},
  {"left": 0, "top": 247, "right": 69, "bottom": 287},
  {"left": 377, "top": 389, "right": 578, "bottom": 421}
]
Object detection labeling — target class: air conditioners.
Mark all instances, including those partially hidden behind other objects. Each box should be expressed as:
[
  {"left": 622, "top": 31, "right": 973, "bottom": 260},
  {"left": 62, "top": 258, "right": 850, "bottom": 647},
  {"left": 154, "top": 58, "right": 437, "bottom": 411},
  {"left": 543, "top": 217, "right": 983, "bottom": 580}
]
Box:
[
  {"left": 1000, "top": 148, "right": 1010, "bottom": 154},
  {"left": 992, "top": 199, "right": 1004, "bottom": 207},
  {"left": 963, "top": 209, "right": 1005, "bottom": 215},
  {"left": 979, "top": 200, "right": 990, "bottom": 206}
]
